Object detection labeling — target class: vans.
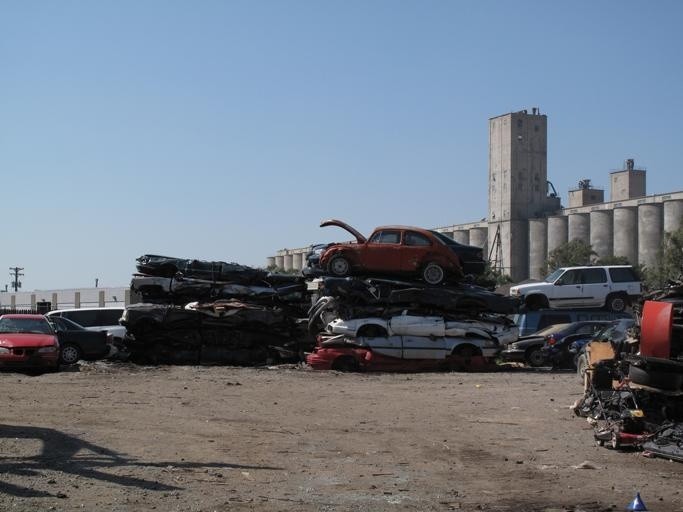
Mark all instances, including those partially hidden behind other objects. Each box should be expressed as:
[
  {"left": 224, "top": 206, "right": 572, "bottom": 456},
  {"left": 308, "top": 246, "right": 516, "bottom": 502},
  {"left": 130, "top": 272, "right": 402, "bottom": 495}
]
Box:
[{"left": 44, "top": 305, "right": 127, "bottom": 339}]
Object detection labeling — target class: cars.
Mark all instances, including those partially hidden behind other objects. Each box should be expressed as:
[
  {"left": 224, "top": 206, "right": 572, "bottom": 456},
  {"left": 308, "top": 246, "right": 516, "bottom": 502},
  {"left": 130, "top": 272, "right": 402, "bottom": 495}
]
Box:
[
  {"left": 502, "top": 319, "right": 613, "bottom": 373},
  {"left": 308, "top": 217, "right": 487, "bottom": 286},
  {"left": 0, "top": 313, "right": 60, "bottom": 371},
  {"left": 509, "top": 264, "right": 643, "bottom": 314},
  {"left": 47, "top": 316, "right": 108, "bottom": 364}
]
